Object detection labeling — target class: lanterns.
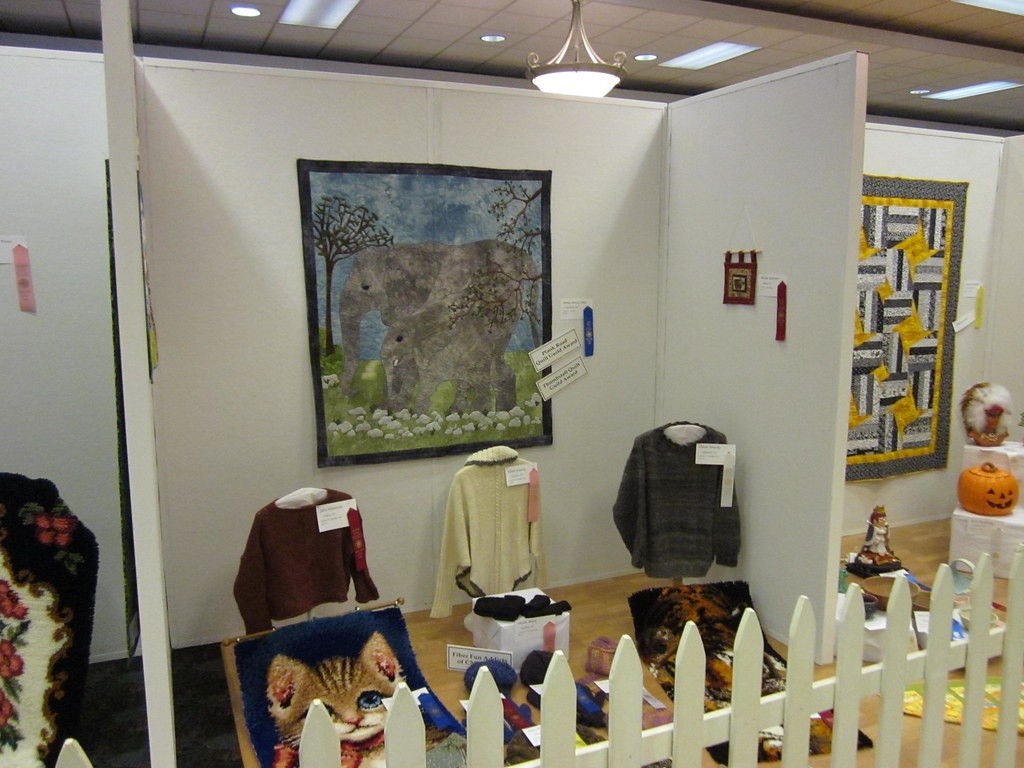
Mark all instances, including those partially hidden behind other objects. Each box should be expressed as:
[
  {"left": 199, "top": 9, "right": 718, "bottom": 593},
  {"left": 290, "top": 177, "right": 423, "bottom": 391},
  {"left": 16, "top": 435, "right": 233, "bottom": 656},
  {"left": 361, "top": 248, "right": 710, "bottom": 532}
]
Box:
[{"left": 957, "top": 462, "right": 1019, "bottom": 514}]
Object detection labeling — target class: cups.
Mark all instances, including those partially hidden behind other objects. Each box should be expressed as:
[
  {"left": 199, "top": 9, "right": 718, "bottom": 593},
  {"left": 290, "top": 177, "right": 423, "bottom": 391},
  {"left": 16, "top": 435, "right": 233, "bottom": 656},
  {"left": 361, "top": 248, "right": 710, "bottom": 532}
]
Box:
[{"left": 862, "top": 593, "right": 878, "bottom": 620}]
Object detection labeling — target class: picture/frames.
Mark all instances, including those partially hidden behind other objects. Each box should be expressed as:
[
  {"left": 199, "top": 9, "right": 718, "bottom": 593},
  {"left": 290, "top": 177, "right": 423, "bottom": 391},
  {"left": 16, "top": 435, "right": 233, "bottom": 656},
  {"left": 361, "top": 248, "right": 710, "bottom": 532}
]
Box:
[{"left": 296, "top": 159, "right": 553, "bottom": 470}]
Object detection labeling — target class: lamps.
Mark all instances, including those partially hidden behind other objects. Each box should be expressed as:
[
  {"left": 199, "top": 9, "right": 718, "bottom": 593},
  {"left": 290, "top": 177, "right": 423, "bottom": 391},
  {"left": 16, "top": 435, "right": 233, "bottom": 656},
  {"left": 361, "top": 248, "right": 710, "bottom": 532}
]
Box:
[{"left": 525, "top": 0, "right": 628, "bottom": 98}]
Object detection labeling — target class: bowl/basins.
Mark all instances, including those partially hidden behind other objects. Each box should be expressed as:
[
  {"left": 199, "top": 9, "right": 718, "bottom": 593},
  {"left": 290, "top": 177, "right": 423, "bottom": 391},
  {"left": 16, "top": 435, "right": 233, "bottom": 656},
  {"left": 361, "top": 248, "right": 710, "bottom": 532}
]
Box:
[
  {"left": 959, "top": 607, "right": 998, "bottom": 630},
  {"left": 859, "top": 575, "right": 922, "bottom": 612},
  {"left": 911, "top": 590, "right": 931, "bottom": 617}
]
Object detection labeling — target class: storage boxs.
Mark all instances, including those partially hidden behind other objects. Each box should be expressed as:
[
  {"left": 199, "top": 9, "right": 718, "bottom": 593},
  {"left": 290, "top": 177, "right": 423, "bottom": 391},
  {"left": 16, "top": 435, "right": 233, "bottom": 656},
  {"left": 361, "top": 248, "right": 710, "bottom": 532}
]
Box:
[{"left": 471, "top": 587, "right": 570, "bottom": 674}]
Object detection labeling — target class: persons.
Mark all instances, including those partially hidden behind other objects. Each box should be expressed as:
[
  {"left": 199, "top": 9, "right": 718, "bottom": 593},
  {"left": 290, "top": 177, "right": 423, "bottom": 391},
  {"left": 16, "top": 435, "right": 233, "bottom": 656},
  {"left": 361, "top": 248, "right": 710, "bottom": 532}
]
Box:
[
  {"left": 961, "top": 382, "right": 1014, "bottom": 447},
  {"left": 864, "top": 505, "right": 892, "bottom": 556}
]
